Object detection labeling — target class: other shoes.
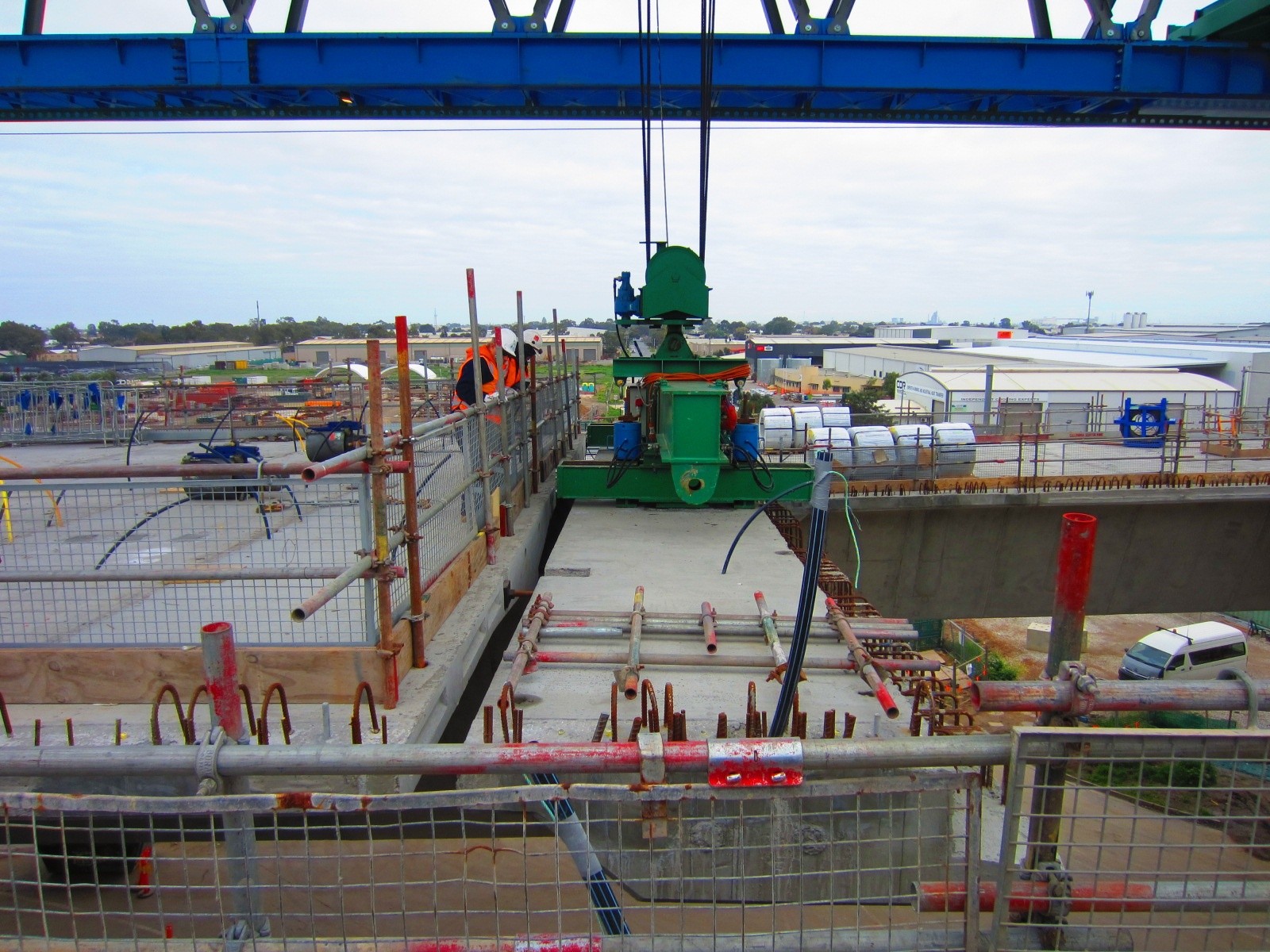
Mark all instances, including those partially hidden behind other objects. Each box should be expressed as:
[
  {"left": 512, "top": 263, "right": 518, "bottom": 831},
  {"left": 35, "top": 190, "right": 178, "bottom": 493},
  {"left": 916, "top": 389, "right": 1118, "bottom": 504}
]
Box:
[{"left": 461, "top": 514, "right": 467, "bottom": 524}]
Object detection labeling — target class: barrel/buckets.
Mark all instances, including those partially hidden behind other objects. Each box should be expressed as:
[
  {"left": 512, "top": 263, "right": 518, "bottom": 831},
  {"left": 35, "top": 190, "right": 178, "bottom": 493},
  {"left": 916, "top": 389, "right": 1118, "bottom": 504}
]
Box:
[
  {"left": 613, "top": 421, "right": 641, "bottom": 461},
  {"left": 732, "top": 423, "right": 759, "bottom": 462}
]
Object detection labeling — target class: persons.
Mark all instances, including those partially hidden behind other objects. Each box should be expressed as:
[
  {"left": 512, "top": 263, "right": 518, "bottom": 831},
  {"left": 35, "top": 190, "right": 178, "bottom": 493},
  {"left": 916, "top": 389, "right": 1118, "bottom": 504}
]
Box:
[
  {"left": 452, "top": 328, "right": 518, "bottom": 525},
  {"left": 504, "top": 329, "right": 543, "bottom": 485}
]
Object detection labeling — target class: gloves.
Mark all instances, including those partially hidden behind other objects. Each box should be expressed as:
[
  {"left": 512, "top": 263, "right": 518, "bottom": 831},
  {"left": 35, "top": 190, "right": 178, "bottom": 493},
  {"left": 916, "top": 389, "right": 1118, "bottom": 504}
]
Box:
[
  {"left": 504, "top": 387, "right": 517, "bottom": 400},
  {"left": 483, "top": 391, "right": 509, "bottom": 404}
]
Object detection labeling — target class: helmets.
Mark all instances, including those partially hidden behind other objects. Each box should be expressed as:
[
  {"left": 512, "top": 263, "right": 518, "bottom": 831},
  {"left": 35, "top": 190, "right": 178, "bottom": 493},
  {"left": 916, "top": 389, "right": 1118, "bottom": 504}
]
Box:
[
  {"left": 493, "top": 328, "right": 518, "bottom": 358},
  {"left": 522, "top": 328, "right": 542, "bottom": 353}
]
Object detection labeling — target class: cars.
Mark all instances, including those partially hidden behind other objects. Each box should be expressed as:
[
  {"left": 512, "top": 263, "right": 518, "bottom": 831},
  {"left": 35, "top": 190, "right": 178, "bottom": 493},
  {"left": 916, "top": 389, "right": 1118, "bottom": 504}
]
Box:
[{"left": 750, "top": 387, "right": 802, "bottom": 401}]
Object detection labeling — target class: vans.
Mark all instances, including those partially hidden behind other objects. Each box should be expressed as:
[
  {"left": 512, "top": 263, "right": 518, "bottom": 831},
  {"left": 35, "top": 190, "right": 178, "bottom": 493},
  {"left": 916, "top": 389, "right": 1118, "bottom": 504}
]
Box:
[{"left": 1118, "top": 620, "right": 1249, "bottom": 683}]
